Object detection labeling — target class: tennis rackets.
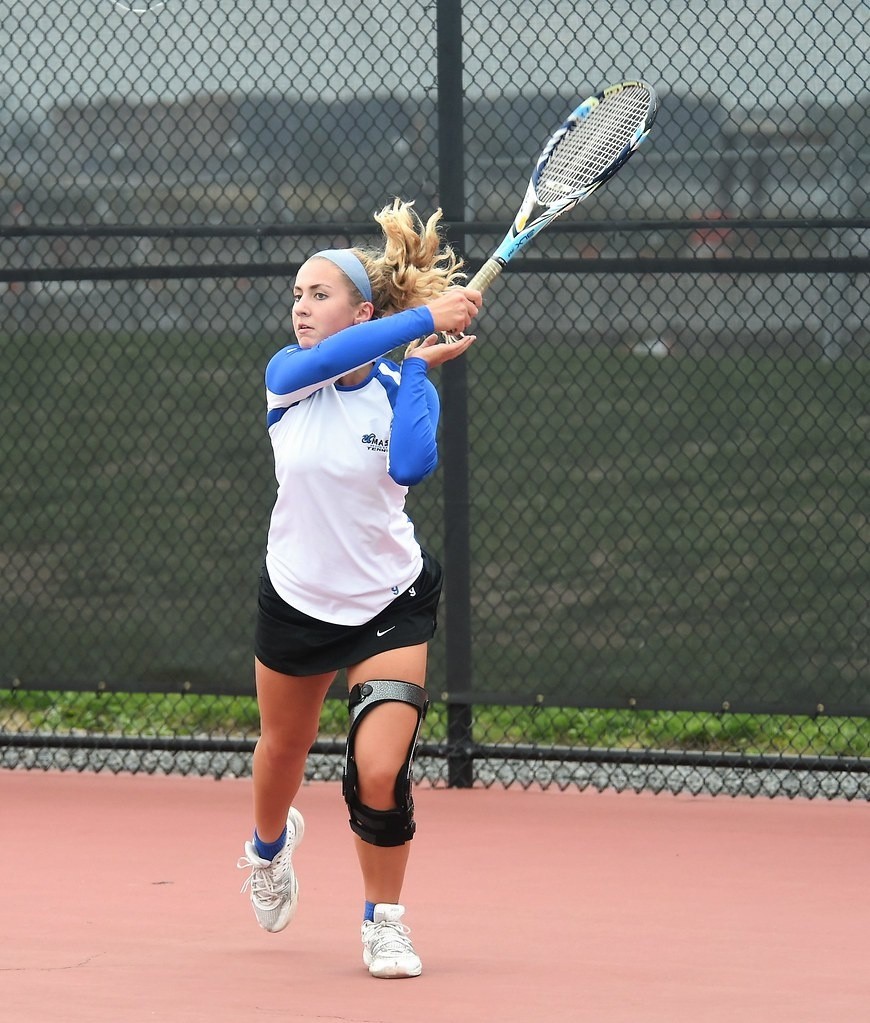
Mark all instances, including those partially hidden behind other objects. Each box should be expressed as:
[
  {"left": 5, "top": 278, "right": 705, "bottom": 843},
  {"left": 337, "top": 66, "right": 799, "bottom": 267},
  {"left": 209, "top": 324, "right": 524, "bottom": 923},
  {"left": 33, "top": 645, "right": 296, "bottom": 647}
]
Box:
[{"left": 446, "top": 78, "right": 659, "bottom": 333}]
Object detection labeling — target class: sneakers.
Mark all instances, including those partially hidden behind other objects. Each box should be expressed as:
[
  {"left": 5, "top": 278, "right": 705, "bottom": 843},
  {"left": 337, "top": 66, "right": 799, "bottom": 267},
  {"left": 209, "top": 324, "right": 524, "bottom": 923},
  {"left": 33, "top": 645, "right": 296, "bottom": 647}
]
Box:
[
  {"left": 236, "top": 804, "right": 304, "bottom": 932},
  {"left": 360, "top": 905, "right": 422, "bottom": 979}
]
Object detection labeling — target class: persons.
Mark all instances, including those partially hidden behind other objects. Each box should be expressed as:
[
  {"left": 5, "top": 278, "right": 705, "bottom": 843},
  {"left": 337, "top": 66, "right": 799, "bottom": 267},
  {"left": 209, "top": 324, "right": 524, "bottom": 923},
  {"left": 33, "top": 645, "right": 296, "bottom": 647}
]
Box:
[{"left": 242, "top": 204, "right": 482, "bottom": 979}]
